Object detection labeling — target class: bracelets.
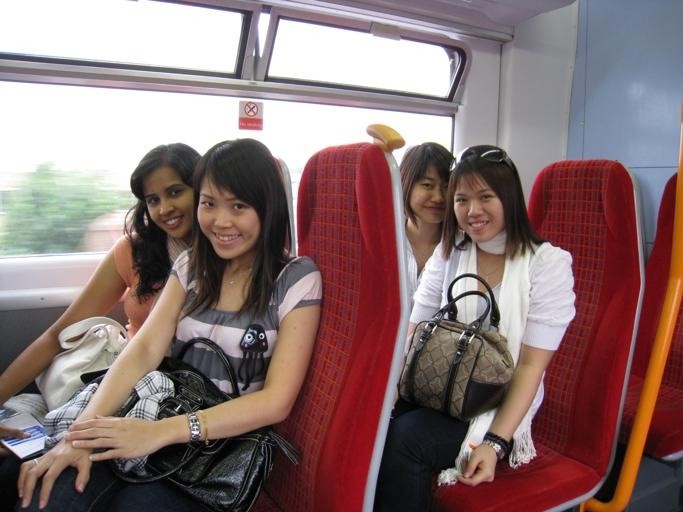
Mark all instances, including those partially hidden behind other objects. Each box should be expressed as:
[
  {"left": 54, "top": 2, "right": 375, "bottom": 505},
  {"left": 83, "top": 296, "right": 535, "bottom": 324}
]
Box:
[
  {"left": 483, "top": 439, "right": 506, "bottom": 461},
  {"left": 199, "top": 409, "right": 209, "bottom": 446},
  {"left": 484, "top": 432, "right": 510, "bottom": 453},
  {"left": 185, "top": 411, "right": 201, "bottom": 444}
]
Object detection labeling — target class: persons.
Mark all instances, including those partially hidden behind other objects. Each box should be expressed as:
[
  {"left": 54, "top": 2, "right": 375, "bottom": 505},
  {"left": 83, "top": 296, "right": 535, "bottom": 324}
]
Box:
[
  {"left": 373, "top": 143, "right": 577, "bottom": 511},
  {"left": 13, "top": 137, "right": 324, "bottom": 511},
  {"left": 397, "top": 142, "right": 457, "bottom": 309},
  {"left": 0, "top": 144, "right": 203, "bottom": 466}
]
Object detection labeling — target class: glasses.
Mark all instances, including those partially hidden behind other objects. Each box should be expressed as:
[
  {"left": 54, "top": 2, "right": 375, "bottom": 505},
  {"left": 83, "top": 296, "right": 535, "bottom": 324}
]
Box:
[{"left": 444, "top": 143, "right": 515, "bottom": 173}]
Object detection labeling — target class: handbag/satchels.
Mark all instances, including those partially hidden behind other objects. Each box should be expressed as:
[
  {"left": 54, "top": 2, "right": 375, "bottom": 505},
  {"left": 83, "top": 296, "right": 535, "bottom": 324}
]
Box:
[
  {"left": 392, "top": 271, "right": 517, "bottom": 425},
  {"left": 93, "top": 334, "right": 304, "bottom": 512}
]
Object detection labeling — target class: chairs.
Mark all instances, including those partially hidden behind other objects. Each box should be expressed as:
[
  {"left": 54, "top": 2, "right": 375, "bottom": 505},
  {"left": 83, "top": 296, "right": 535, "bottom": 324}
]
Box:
[
  {"left": 266, "top": 152, "right": 299, "bottom": 258},
  {"left": 616, "top": 168, "right": 682, "bottom": 463},
  {"left": 250, "top": 139, "right": 413, "bottom": 512},
  {"left": 427, "top": 157, "right": 649, "bottom": 512}
]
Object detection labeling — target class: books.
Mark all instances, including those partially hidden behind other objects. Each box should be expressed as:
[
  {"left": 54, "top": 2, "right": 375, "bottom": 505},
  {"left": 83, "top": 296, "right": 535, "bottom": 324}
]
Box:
[{"left": 1, "top": 412, "right": 48, "bottom": 461}]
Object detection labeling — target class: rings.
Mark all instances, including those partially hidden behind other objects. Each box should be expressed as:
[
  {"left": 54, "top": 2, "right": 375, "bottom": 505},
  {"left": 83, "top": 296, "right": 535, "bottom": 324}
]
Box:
[{"left": 33, "top": 458, "right": 39, "bottom": 466}]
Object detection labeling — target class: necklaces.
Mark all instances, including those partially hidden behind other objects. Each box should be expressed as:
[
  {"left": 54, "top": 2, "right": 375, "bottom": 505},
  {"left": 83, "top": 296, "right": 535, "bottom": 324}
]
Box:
[
  {"left": 412, "top": 240, "right": 438, "bottom": 260},
  {"left": 478, "top": 260, "right": 499, "bottom": 284},
  {"left": 164, "top": 233, "right": 191, "bottom": 267},
  {"left": 222, "top": 264, "right": 253, "bottom": 287}
]
